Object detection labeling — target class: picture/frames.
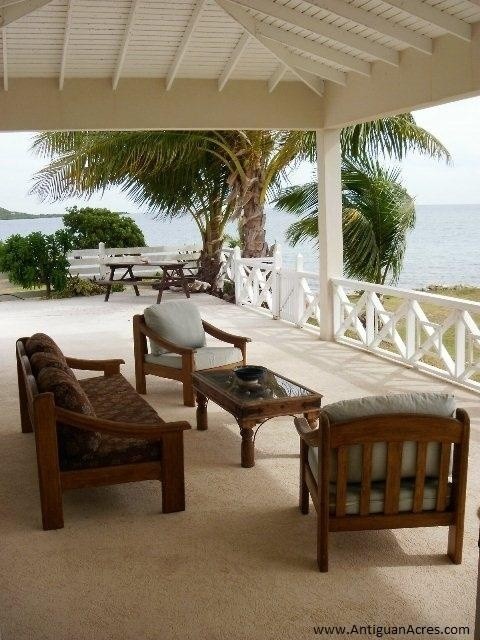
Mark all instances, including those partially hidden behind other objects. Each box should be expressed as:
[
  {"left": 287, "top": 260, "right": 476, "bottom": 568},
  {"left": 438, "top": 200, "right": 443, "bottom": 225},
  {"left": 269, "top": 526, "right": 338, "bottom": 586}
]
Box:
[{"left": 104, "top": 261, "right": 191, "bottom": 304}]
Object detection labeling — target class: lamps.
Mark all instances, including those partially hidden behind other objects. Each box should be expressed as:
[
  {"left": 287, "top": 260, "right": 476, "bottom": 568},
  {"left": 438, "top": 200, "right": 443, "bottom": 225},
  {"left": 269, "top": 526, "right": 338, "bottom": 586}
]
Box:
[
  {"left": 132, "top": 313, "right": 252, "bottom": 407},
  {"left": 293, "top": 408, "right": 471, "bottom": 573}
]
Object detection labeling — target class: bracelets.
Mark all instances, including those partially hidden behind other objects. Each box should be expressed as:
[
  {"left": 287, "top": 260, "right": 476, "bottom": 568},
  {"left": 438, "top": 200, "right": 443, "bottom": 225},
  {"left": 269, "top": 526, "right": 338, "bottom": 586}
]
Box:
[{"left": 234, "top": 368, "right": 264, "bottom": 382}]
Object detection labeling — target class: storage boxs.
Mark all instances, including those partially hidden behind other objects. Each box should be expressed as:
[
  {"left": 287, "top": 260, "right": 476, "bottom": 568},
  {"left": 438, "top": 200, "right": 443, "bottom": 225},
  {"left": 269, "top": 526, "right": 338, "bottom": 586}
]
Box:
[
  {"left": 97, "top": 281, "right": 171, "bottom": 286},
  {"left": 16, "top": 337, "right": 191, "bottom": 531},
  {"left": 134, "top": 276, "right": 199, "bottom": 279}
]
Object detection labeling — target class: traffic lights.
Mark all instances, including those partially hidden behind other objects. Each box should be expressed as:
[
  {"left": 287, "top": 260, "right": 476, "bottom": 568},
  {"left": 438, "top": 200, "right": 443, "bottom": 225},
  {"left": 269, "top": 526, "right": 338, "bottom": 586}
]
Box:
[
  {"left": 323, "top": 391, "right": 456, "bottom": 486},
  {"left": 25, "top": 333, "right": 102, "bottom": 462},
  {"left": 144, "top": 301, "right": 206, "bottom": 356}
]
